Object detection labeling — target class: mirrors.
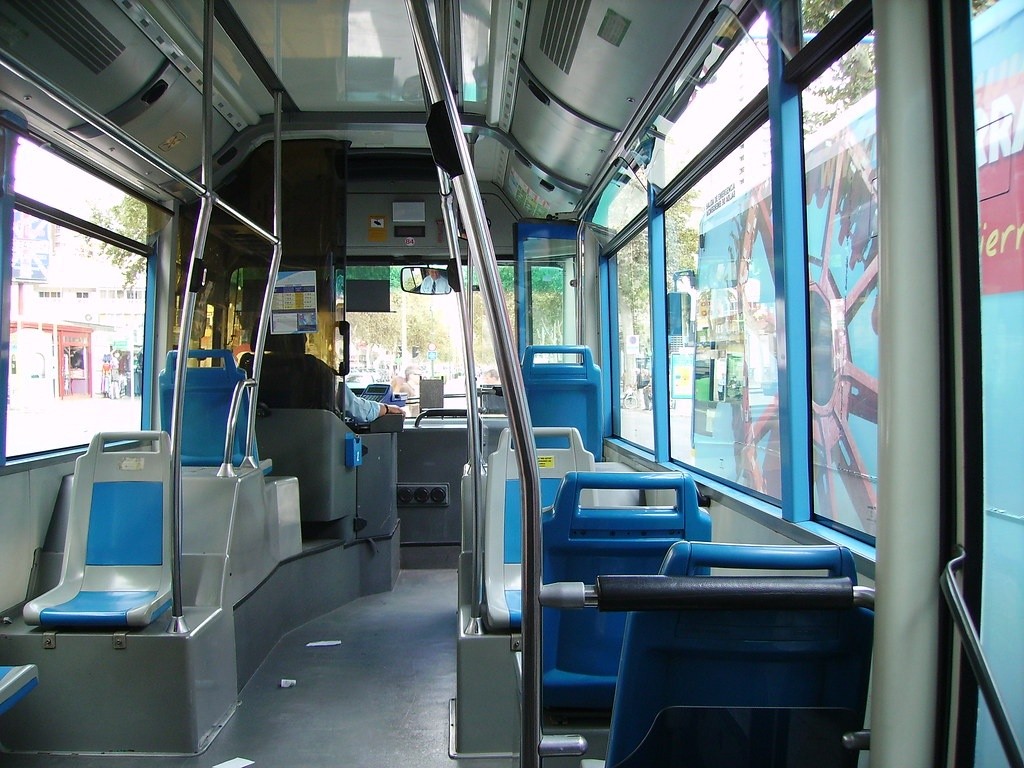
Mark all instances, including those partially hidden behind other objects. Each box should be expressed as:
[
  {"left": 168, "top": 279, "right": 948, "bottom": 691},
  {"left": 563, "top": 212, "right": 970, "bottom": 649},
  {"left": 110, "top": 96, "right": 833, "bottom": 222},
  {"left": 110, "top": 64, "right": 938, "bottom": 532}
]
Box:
[{"left": 401, "top": 266, "right": 453, "bottom": 295}]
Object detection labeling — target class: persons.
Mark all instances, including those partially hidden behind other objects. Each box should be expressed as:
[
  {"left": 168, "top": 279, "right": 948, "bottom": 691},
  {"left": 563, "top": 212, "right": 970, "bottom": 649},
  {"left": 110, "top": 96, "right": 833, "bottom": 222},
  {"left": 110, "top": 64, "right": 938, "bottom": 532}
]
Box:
[
  {"left": 633, "top": 367, "right": 651, "bottom": 410},
  {"left": 303, "top": 333, "right": 407, "bottom": 423},
  {"left": 420, "top": 269, "right": 450, "bottom": 294},
  {"left": 100, "top": 348, "right": 127, "bottom": 398}
]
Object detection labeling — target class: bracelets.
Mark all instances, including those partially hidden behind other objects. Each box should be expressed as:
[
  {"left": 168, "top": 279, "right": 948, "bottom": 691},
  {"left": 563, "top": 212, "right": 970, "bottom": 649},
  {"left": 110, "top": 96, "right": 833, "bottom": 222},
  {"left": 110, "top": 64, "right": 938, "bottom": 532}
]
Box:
[{"left": 383, "top": 403, "right": 389, "bottom": 416}]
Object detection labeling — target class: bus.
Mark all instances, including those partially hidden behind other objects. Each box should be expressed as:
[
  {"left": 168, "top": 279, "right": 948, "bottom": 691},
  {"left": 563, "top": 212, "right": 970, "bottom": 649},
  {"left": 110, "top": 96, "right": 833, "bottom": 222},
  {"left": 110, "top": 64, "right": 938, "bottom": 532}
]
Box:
[{"left": 665, "top": 0, "right": 1024, "bottom": 768}]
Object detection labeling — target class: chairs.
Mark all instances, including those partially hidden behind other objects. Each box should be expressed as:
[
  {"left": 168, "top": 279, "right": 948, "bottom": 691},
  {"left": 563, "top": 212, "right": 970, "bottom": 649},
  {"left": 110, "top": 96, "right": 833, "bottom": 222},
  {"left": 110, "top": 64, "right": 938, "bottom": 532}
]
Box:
[
  {"left": 237, "top": 316, "right": 347, "bottom": 419},
  {"left": 573, "top": 534, "right": 875, "bottom": 767},
  {"left": 484, "top": 426, "right": 598, "bottom": 634},
  {"left": 511, "top": 470, "right": 714, "bottom": 711},
  {"left": 158, "top": 349, "right": 276, "bottom": 480},
  {"left": 21, "top": 430, "right": 178, "bottom": 628},
  {"left": 520, "top": 343, "right": 606, "bottom": 465}
]
